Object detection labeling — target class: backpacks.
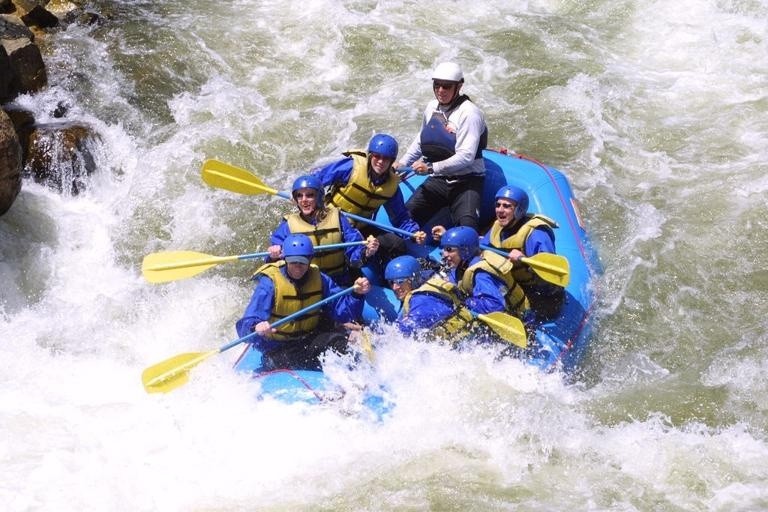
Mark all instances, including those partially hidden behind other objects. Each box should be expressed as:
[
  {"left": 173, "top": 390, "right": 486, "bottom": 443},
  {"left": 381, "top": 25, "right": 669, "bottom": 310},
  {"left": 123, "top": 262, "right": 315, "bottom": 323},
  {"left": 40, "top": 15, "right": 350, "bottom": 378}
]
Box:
[{"left": 421, "top": 107, "right": 457, "bottom": 164}]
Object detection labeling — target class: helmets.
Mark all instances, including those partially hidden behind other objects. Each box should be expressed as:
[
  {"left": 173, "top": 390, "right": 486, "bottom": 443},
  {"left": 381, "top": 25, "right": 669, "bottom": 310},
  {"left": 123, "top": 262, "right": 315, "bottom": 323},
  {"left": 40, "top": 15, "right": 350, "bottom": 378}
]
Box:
[
  {"left": 440, "top": 226, "right": 479, "bottom": 260},
  {"left": 495, "top": 185, "right": 529, "bottom": 220},
  {"left": 385, "top": 256, "right": 420, "bottom": 279},
  {"left": 282, "top": 233, "right": 315, "bottom": 259},
  {"left": 368, "top": 135, "right": 398, "bottom": 159},
  {"left": 432, "top": 62, "right": 463, "bottom": 81},
  {"left": 292, "top": 176, "right": 325, "bottom": 193}
]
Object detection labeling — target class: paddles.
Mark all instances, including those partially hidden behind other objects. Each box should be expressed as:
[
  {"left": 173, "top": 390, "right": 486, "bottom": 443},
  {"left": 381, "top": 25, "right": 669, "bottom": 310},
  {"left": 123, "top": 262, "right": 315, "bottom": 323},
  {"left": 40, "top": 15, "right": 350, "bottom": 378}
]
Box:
[
  {"left": 202, "top": 159, "right": 421, "bottom": 238},
  {"left": 143, "top": 283, "right": 361, "bottom": 394},
  {"left": 141, "top": 241, "right": 368, "bottom": 283},
  {"left": 435, "top": 232, "right": 570, "bottom": 287},
  {"left": 467, "top": 311, "right": 527, "bottom": 349}
]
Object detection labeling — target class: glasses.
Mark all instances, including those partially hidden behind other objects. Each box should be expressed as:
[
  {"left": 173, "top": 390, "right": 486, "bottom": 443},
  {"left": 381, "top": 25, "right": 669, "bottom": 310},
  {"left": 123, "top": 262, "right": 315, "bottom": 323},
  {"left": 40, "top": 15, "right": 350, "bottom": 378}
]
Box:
[
  {"left": 389, "top": 280, "right": 404, "bottom": 285},
  {"left": 441, "top": 247, "right": 459, "bottom": 252},
  {"left": 434, "top": 82, "right": 454, "bottom": 90},
  {"left": 371, "top": 153, "right": 393, "bottom": 161},
  {"left": 496, "top": 203, "right": 512, "bottom": 208},
  {"left": 296, "top": 194, "right": 316, "bottom": 197}
]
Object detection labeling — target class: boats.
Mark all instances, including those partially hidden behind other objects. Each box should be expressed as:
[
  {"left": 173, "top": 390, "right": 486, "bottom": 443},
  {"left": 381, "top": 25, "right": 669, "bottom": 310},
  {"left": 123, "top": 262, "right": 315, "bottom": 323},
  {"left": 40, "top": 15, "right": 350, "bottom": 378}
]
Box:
[{"left": 229, "top": 149, "right": 605, "bottom": 430}]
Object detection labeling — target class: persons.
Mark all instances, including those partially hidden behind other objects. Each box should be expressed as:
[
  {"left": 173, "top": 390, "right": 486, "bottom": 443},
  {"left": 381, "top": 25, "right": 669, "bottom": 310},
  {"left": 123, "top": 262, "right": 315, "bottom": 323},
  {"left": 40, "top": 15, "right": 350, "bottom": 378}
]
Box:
[
  {"left": 439, "top": 225, "right": 532, "bottom": 319},
  {"left": 291, "top": 133, "right": 427, "bottom": 246},
  {"left": 232, "top": 234, "right": 370, "bottom": 376},
  {"left": 344, "top": 255, "right": 489, "bottom": 356},
  {"left": 265, "top": 176, "right": 383, "bottom": 325},
  {"left": 390, "top": 63, "right": 488, "bottom": 233},
  {"left": 430, "top": 186, "right": 567, "bottom": 320}
]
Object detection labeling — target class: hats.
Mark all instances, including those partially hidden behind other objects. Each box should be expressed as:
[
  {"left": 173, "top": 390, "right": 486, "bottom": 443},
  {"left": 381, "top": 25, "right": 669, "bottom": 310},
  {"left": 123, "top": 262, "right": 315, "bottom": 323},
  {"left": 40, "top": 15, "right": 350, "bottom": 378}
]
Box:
[{"left": 284, "top": 256, "right": 311, "bottom": 266}]
